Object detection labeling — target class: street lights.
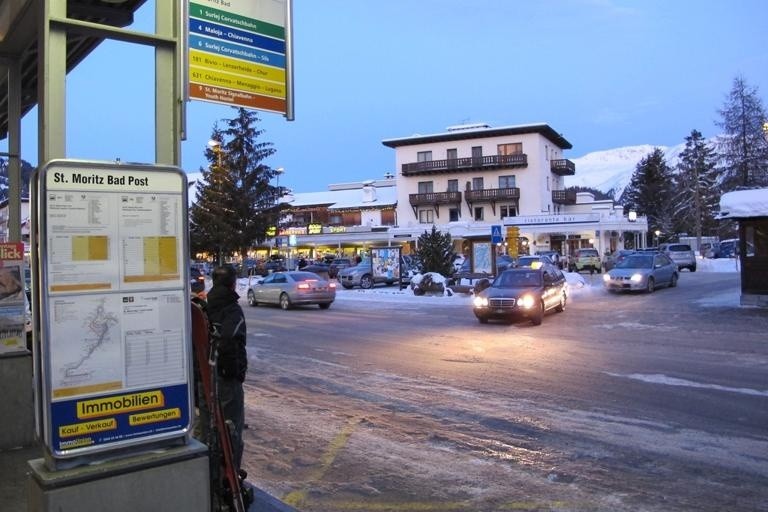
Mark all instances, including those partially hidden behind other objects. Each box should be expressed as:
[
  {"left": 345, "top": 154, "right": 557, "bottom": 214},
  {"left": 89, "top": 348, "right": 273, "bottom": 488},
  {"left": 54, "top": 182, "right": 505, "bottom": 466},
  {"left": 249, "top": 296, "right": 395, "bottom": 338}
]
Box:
[
  {"left": 277, "top": 168, "right": 285, "bottom": 247},
  {"left": 208, "top": 140, "right": 222, "bottom": 267},
  {"left": 655, "top": 230, "right": 660, "bottom": 246}
]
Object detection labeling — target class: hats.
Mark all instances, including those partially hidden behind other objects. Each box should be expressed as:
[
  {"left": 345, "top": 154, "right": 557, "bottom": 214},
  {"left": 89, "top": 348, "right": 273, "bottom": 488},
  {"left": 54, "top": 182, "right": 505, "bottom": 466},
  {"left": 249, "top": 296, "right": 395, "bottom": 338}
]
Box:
[{"left": 190, "top": 268, "right": 205, "bottom": 293}]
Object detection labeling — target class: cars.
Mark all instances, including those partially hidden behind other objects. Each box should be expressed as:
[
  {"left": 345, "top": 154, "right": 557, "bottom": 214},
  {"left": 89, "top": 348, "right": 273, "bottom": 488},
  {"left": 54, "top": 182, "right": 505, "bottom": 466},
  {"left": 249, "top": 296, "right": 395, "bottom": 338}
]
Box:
[
  {"left": 605, "top": 249, "right": 636, "bottom": 271},
  {"left": 602, "top": 251, "right": 680, "bottom": 294},
  {"left": 24, "top": 269, "right": 31, "bottom": 301},
  {"left": 701, "top": 238, "right": 742, "bottom": 259},
  {"left": 337, "top": 258, "right": 396, "bottom": 289},
  {"left": 497, "top": 251, "right": 568, "bottom": 276},
  {"left": 661, "top": 243, "right": 697, "bottom": 271},
  {"left": 246, "top": 271, "right": 337, "bottom": 310},
  {"left": 190, "top": 257, "right": 358, "bottom": 282},
  {"left": 570, "top": 247, "right": 602, "bottom": 274},
  {"left": 473, "top": 266, "right": 570, "bottom": 326}
]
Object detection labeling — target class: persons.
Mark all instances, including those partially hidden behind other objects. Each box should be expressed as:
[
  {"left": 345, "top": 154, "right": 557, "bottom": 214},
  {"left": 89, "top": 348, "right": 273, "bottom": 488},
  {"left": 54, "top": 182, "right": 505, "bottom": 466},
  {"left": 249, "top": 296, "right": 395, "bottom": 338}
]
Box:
[{"left": 190, "top": 266, "right": 253, "bottom": 504}]
